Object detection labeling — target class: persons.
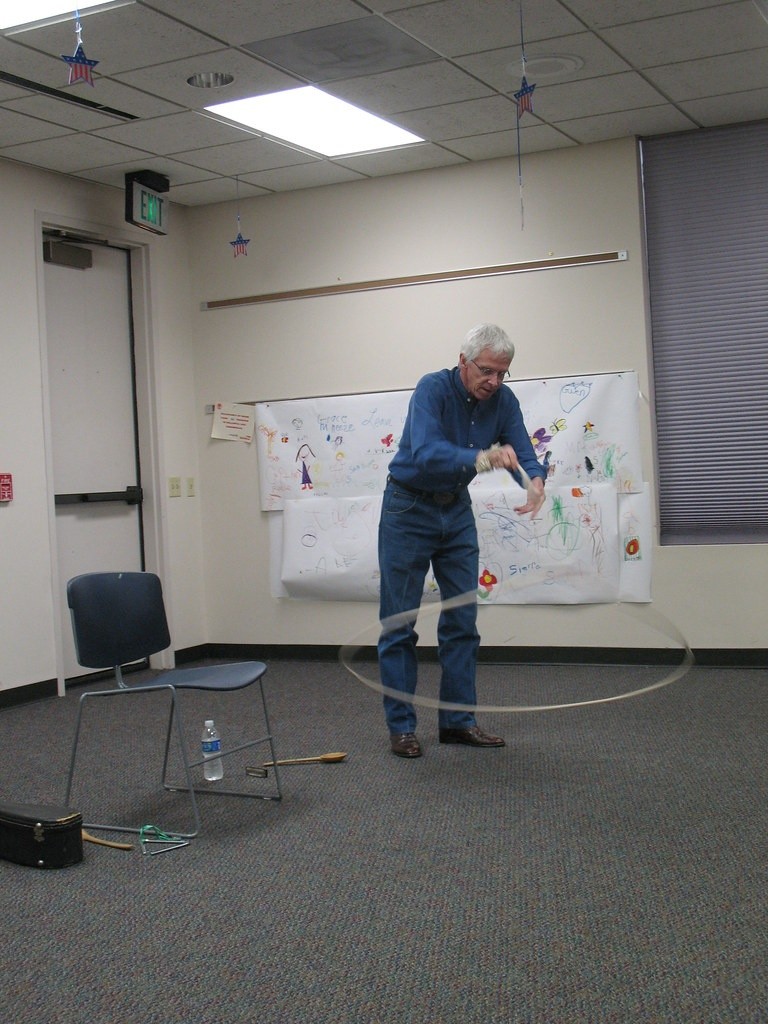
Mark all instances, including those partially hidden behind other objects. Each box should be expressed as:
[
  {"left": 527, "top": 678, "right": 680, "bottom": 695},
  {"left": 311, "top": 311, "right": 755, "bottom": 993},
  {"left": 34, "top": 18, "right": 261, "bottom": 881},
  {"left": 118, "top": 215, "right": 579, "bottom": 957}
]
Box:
[{"left": 378, "top": 323, "right": 547, "bottom": 758}]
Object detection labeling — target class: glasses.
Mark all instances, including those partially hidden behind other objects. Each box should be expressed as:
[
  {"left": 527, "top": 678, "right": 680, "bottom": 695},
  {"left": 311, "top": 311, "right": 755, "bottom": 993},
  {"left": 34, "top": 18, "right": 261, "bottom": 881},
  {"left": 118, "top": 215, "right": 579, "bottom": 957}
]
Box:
[{"left": 471, "top": 358, "right": 511, "bottom": 380}]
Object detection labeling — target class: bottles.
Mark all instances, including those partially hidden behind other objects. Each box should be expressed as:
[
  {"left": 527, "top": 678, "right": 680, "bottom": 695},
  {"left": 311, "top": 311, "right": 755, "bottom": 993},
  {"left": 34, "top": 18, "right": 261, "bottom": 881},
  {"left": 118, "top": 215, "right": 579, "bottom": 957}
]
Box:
[{"left": 202, "top": 720, "right": 223, "bottom": 780}]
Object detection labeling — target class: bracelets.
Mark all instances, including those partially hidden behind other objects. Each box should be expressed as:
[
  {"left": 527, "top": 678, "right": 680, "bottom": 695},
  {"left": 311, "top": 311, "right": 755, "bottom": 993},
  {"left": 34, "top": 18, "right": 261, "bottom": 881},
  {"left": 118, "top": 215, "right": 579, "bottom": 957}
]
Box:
[{"left": 476, "top": 442, "right": 500, "bottom": 473}]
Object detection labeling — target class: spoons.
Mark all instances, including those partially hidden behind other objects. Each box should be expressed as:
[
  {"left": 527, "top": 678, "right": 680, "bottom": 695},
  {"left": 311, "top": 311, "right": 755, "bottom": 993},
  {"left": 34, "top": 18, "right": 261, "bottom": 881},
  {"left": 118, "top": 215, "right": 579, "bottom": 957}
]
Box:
[
  {"left": 82, "top": 828, "right": 132, "bottom": 848},
  {"left": 262, "top": 752, "right": 347, "bottom": 765}
]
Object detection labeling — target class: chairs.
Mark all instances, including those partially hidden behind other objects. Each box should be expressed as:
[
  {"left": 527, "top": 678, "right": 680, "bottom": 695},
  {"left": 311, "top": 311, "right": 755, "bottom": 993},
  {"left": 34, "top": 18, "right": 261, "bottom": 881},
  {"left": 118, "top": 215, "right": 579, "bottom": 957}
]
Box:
[{"left": 65, "top": 570, "right": 289, "bottom": 838}]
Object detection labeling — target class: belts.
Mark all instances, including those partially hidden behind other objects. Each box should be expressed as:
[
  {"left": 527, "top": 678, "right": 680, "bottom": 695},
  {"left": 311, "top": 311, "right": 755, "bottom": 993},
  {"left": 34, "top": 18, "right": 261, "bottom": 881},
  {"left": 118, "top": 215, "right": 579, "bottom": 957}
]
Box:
[{"left": 391, "top": 477, "right": 466, "bottom": 505}]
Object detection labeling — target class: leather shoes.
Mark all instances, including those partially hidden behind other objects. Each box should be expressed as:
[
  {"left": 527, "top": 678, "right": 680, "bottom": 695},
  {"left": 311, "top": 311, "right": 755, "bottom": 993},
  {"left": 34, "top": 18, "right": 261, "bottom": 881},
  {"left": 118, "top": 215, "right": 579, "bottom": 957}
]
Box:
[
  {"left": 391, "top": 731, "right": 421, "bottom": 757},
  {"left": 439, "top": 725, "right": 505, "bottom": 746}
]
